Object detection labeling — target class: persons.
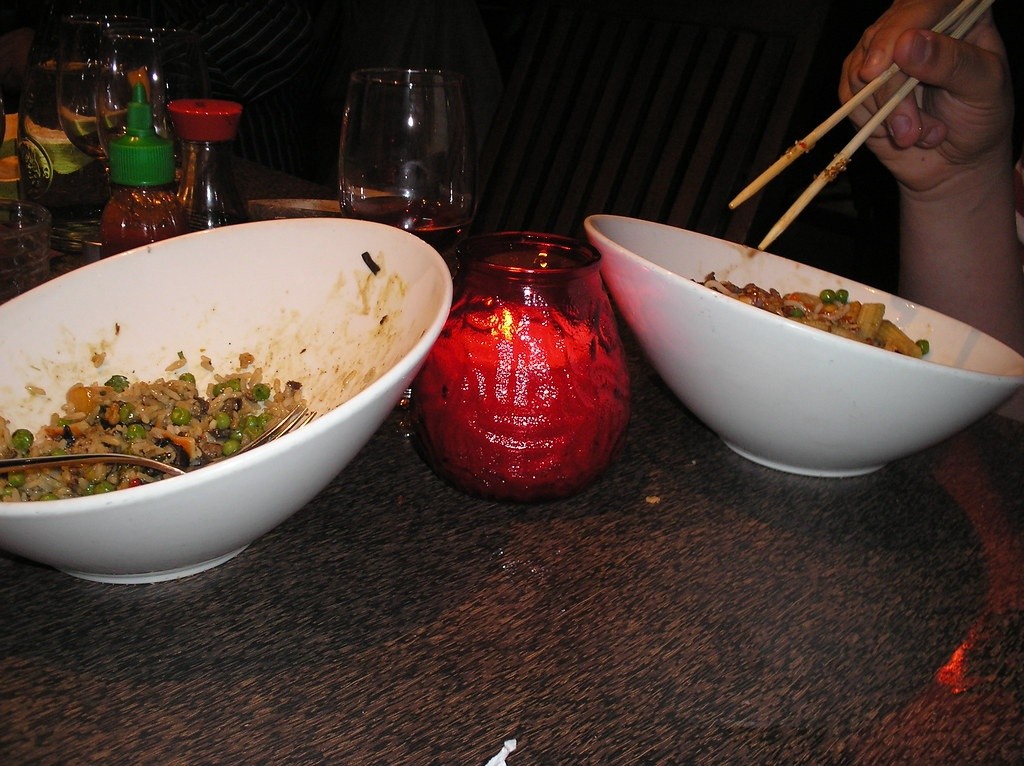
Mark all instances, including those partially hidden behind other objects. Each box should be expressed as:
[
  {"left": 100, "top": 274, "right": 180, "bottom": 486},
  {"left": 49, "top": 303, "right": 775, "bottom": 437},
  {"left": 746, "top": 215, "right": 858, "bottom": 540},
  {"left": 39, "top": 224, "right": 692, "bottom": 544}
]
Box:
[{"left": 838, "top": 0, "right": 1024, "bottom": 422}]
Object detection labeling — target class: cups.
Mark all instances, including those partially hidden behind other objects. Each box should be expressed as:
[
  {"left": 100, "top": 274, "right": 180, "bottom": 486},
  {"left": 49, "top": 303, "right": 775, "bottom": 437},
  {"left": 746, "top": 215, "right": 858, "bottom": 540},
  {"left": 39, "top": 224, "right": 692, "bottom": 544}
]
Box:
[{"left": 0, "top": 197, "right": 51, "bottom": 304}]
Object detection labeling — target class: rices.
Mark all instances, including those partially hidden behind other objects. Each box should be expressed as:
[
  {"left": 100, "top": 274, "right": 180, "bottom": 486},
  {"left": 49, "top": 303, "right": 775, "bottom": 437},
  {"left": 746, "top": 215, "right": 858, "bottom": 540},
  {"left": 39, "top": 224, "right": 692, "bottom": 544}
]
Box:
[
  {"left": 692, "top": 271, "right": 930, "bottom": 359},
  {"left": 0, "top": 353, "right": 304, "bottom": 501}
]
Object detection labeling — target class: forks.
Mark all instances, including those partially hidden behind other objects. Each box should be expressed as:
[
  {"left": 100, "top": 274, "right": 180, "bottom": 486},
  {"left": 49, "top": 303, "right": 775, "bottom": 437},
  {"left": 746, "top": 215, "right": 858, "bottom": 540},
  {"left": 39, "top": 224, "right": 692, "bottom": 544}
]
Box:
[{"left": 0, "top": 405, "right": 317, "bottom": 477}]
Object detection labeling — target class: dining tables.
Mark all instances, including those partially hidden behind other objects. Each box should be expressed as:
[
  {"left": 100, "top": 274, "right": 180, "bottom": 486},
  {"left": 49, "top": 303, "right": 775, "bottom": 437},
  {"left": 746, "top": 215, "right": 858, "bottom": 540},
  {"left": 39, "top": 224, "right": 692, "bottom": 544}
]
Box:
[{"left": 0, "top": 151, "right": 1024, "bottom": 766}]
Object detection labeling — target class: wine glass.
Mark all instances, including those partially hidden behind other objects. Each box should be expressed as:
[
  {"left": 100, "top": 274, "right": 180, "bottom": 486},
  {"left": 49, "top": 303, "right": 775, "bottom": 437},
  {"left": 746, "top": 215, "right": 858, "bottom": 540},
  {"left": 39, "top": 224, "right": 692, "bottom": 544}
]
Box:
[
  {"left": 61, "top": 13, "right": 156, "bottom": 246},
  {"left": 95, "top": 25, "right": 207, "bottom": 200},
  {"left": 340, "top": 69, "right": 480, "bottom": 435}
]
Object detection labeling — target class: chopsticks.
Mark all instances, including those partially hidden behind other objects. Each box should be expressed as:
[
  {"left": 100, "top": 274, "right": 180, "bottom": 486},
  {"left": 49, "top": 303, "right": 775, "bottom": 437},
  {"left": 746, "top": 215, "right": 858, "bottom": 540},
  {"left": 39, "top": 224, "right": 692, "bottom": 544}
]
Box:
[{"left": 729, "top": 0, "right": 997, "bottom": 251}]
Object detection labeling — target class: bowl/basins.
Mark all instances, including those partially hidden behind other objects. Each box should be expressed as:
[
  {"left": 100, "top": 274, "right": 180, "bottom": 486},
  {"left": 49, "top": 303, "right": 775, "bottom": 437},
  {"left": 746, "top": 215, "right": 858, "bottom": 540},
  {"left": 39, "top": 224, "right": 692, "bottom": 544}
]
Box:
[
  {"left": 0, "top": 217, "right": 454, "bottom": 584},
  {"left": 585, "top": 213, "right": 1023, "bottom": 475}
]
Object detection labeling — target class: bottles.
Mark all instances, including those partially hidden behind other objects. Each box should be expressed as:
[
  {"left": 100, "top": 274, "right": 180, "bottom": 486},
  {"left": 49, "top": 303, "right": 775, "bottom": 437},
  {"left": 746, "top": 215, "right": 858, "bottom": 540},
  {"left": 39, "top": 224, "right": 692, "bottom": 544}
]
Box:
[
  {"left": 165, "top": 98, "right": 250, "bottom": 233},
  {"left": 102, "top": 84, "right": 185, "bottom": 258},
  {"left": 15, "top": 0, "right": 124, "bottom": 212},
  {"left": 414, "top": 233, "right": 633, "bottom": 500}
]
